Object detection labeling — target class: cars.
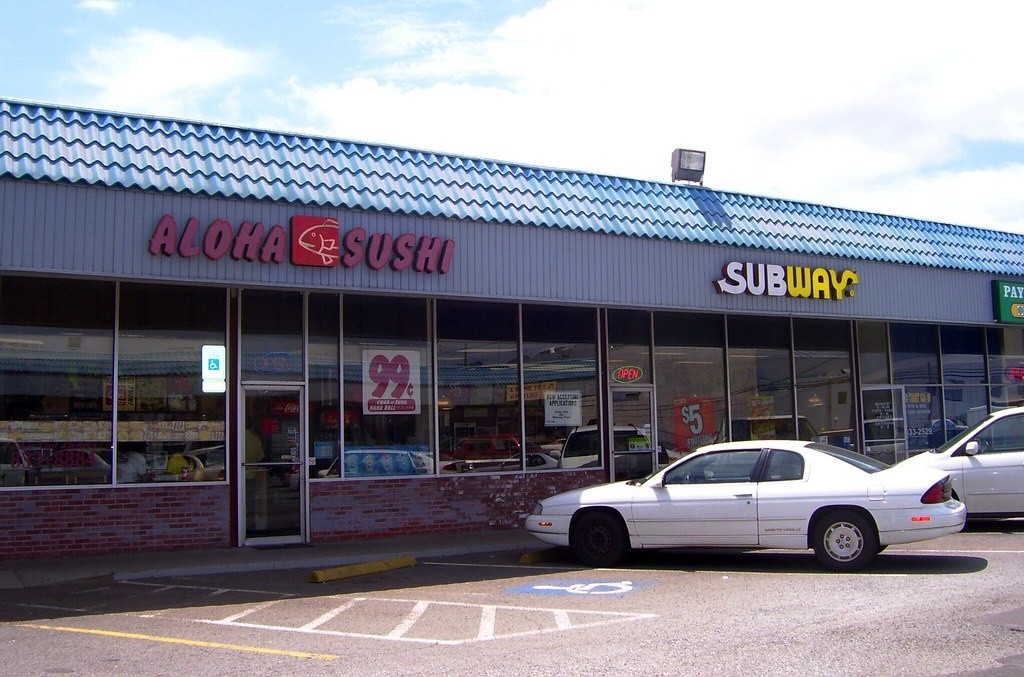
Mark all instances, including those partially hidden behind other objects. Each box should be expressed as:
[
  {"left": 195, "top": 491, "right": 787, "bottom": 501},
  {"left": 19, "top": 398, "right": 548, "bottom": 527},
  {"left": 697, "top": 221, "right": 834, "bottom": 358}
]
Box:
[
  {"left": 0, "top": 404, "right": 980, "bottom": 487},
  {"left": 890, "top": 404, "right": 1024, "bottom": 521}
]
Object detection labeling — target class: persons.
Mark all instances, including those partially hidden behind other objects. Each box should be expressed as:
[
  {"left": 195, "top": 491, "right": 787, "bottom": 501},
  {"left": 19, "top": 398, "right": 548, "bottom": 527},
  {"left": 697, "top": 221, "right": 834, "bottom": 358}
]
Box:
[
  {"left": 245, "top": 415, "right": 266, "bottom": 536},
  {"left": 117, "top": 443, "right": 193, "bottom": 482}
]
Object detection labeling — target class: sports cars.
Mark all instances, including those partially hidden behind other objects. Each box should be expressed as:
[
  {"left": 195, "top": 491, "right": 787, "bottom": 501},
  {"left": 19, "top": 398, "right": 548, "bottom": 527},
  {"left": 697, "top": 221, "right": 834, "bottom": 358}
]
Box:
[{"left": 524, "top": 438, "right": 966, "bottom": 574}]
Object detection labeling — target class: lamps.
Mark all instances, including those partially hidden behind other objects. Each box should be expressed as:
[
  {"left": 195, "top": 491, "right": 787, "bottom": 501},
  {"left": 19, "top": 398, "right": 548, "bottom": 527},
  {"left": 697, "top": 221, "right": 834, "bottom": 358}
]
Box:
[{"left": 670, "top": 147, "right": 706, "bottom": 184}]
